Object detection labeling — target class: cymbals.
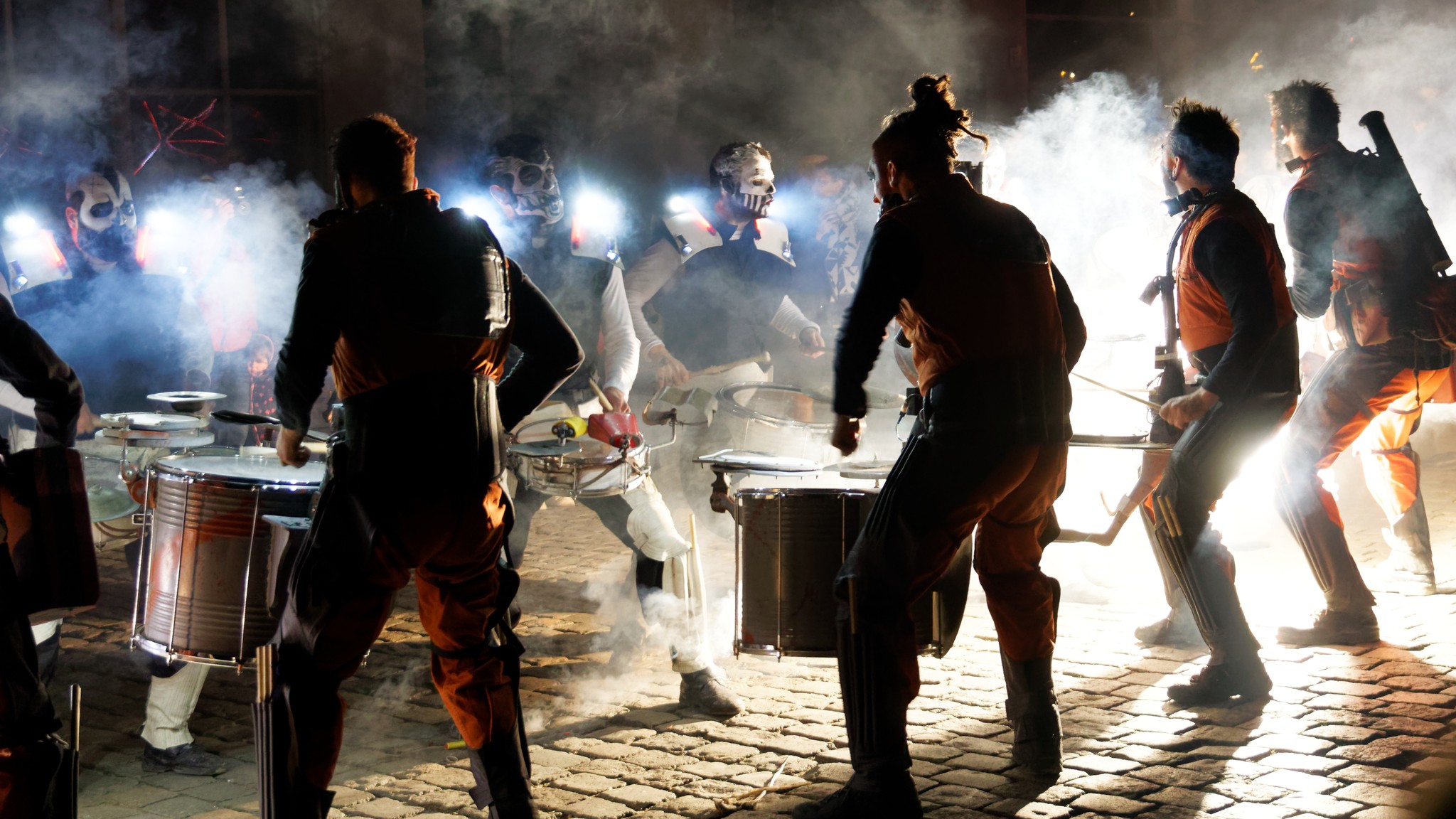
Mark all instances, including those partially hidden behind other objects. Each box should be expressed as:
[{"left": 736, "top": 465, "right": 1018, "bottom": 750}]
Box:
[
  {"left": 801, "top": 381, "right": 907, "bottom": 410},
  {"left": 147, "top": 391, "right": 227, "bottom": 413},
  {"left": 81, "top": 453, "right": 140, "bottom": 521},
  {"left": 1069, "top": 428, "right": 1150, "bottom": 445},
  {"left": 933, "top": 533, "right": 972, "bottom": 660},
  {"left": 821, "top": 460, "right": 896, "bottom": 472}
]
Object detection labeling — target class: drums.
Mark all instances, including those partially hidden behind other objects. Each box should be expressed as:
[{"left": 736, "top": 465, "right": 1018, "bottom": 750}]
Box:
[
  {"left": 135, "top": 453, "right": 326, "bottom": 670},
  {"left": 0, "top": 446, "right": 101, "bottom": 628},
  {"left": 716, "top": 380, "right": 866, "bottom": 461},
  {"left": 734, "top": 487, "right": 939, "bottom": 657},
  {"left": 513, "top": 415, "right": 653, "bottom": 499}
]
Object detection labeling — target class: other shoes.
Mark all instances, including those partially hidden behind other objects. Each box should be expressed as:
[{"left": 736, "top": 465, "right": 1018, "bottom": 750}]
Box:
[
  {"left": 795, "top": 769, "right": 925, "bottom": 819},
  {"left": 1135, "top": 621, "right": 1204, "bottom": 647},
  {"left": 678, "top": 668, "right": 742, "bottom": 714},
  {"left": 1279, "top": 607, "right": 1379, "bottom": 645},
  {"left": 1363, "top": 566, "right": 1438, "bottom": 596},
  {"left": 1168, "top": 651, "right": 1271, "bottom": 704},
  {"left": 1008, "top": 729, "right": 1061, "bottom": 782},
  {"left": 141, "top": 740, "right": 225, "bottom": 776}
]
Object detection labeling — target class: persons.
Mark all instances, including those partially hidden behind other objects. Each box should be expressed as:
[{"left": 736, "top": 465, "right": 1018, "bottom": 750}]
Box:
[
  {"left": 606, "top": 140, "right": 826, "bottom": 675},
  {"left": 1134, "top": 97, "right": 1302, "bottom": 708},
  {"left": 449, "top": 132, "right": 745, "bottom": 736},
  {"left": 788, "top": 74, "right": 1086, "bottom": 819},
  {"left": 12, "top": 163, "right": 228, "bottom": 777},
  {"left": 258, "top": 113, "right": 585, "bottom": 819},
  {"left": 1271, "top": 78, "right": 1456, "bottom": 646},
  {"left": 0, "top": 280, "right": 99, "bottom": 819}
]
